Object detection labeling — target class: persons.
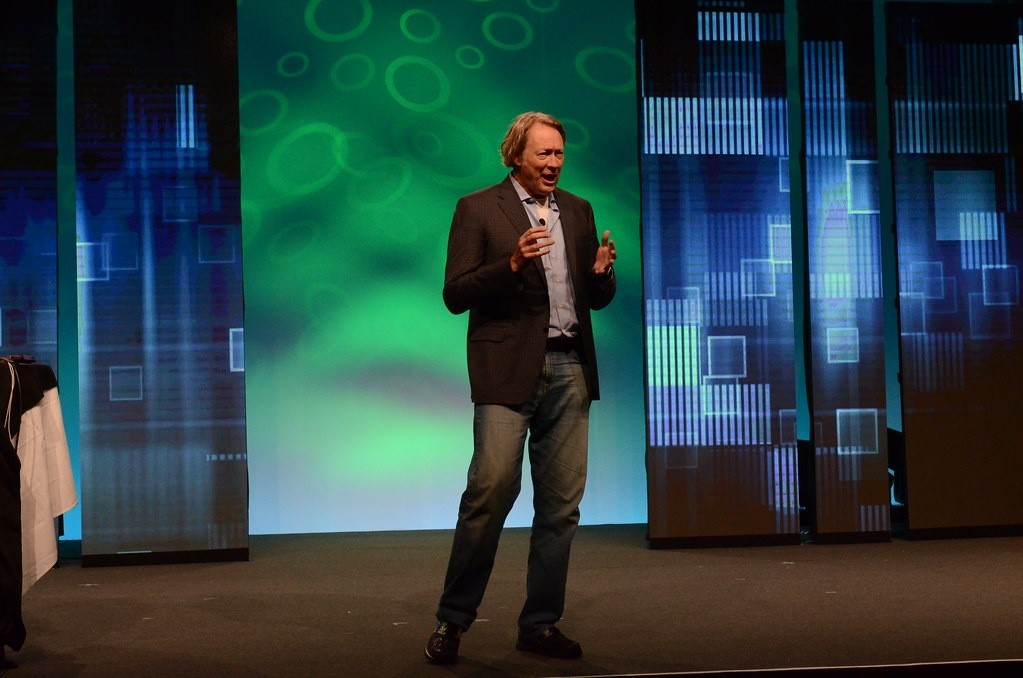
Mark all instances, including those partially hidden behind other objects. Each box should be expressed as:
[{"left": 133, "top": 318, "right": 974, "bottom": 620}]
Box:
[{"left": 423, "top": 110, "right": 618, "bottom": 665}]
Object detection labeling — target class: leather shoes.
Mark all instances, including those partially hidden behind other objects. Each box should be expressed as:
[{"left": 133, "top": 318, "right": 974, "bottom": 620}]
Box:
[
  {"left": 424, "top": 618, "right": 463, "bottom": 663},
  {"left": 516, "top": 626, "right": 582, "bottom": 658}
]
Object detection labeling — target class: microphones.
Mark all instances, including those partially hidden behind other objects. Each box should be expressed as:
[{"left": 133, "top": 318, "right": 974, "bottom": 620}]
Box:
[{"left": 539, "top": 218, "right": 546, "bottom": 225}]
